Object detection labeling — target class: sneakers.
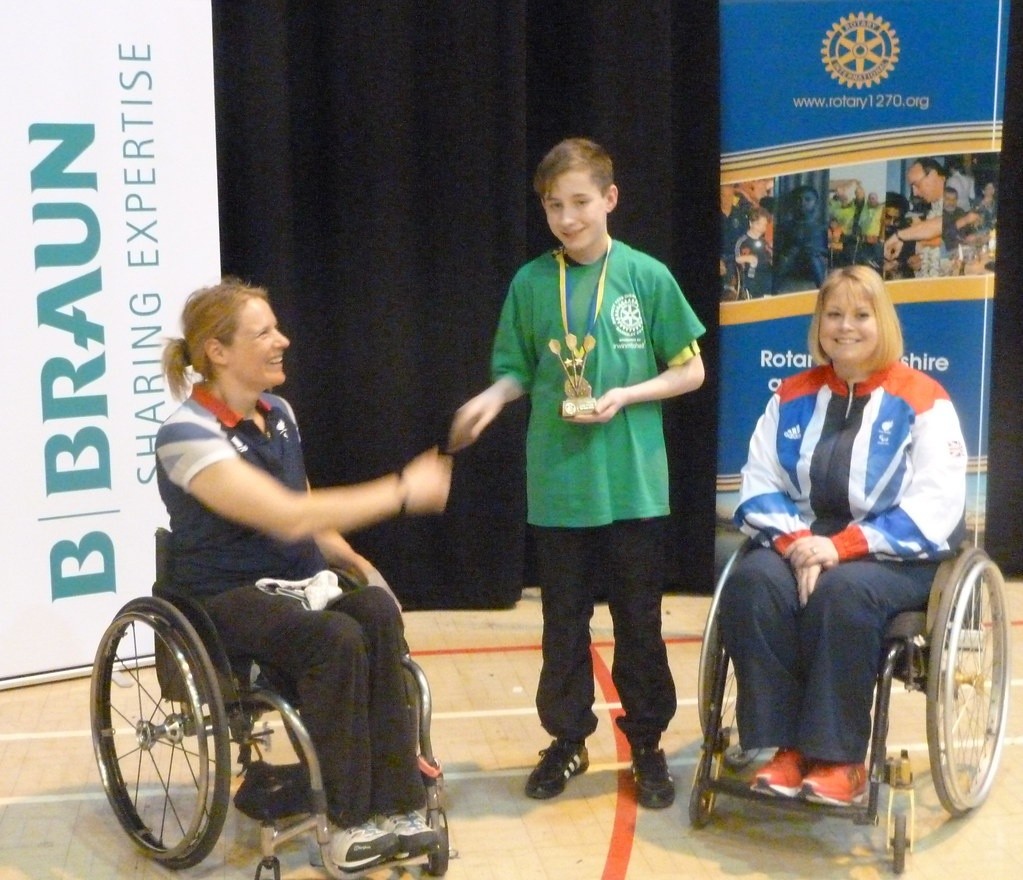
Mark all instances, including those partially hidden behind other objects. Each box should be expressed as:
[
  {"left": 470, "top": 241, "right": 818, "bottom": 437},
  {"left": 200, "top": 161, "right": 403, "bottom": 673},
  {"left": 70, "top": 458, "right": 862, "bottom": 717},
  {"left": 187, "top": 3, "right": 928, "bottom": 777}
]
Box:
[
  {"left": 802, "top": 759, "right": 868, "bottom": 806},
  {"left": 371, "top": 809, "right": 436, "bottom": 860},
  {"left": 525, "top": 740, "right": 590, "bottom": 799},
  {"left": 632, "top": 748, "right": 675, "bottom": 809},
  {"left": 322, "top": 818, "right": 402, "bottom": 873},
  {"left": 748, "top": 745, "right": 808, "bottom": 802}
]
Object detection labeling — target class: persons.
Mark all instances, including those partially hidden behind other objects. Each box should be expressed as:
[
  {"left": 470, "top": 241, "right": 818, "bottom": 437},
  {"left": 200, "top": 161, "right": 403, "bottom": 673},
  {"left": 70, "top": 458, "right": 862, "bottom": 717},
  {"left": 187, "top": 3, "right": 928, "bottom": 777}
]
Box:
[
  {"left": 153, "top": 277, "right": 442, "bottom": 875},
  {"left": 716, "top": 263, "right": 968, "bottom": 806},
  {"left": 445, "top": 136, "right": 706, "bottom": 812},
  {"left": 720, "top": 153, "right": 997, "bottom": 303}
]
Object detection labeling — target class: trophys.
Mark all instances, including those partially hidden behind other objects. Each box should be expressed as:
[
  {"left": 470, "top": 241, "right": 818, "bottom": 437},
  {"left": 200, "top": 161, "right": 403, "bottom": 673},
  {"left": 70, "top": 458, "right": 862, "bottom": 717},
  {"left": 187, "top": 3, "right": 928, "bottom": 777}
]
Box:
[{"left": 549, "top": 333, "right": 597, "bottom": 418}]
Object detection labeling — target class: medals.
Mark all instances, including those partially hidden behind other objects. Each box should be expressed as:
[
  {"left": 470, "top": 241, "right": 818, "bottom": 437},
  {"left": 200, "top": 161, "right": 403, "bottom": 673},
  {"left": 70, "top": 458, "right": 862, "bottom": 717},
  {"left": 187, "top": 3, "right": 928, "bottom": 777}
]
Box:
[{"left": 564, "top": 374, "right": 593, "bottom": 400}]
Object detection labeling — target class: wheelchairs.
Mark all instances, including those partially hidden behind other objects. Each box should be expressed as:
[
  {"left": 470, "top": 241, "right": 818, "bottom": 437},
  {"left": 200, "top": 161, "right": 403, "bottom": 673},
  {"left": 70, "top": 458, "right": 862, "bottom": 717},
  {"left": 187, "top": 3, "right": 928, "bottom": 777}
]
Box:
[
  {"left": 689, "top": 512, "right": 1013, "bottom": 876},
  {"left": 90, "top": 526, "right": 450, "bottom": 880}
]
